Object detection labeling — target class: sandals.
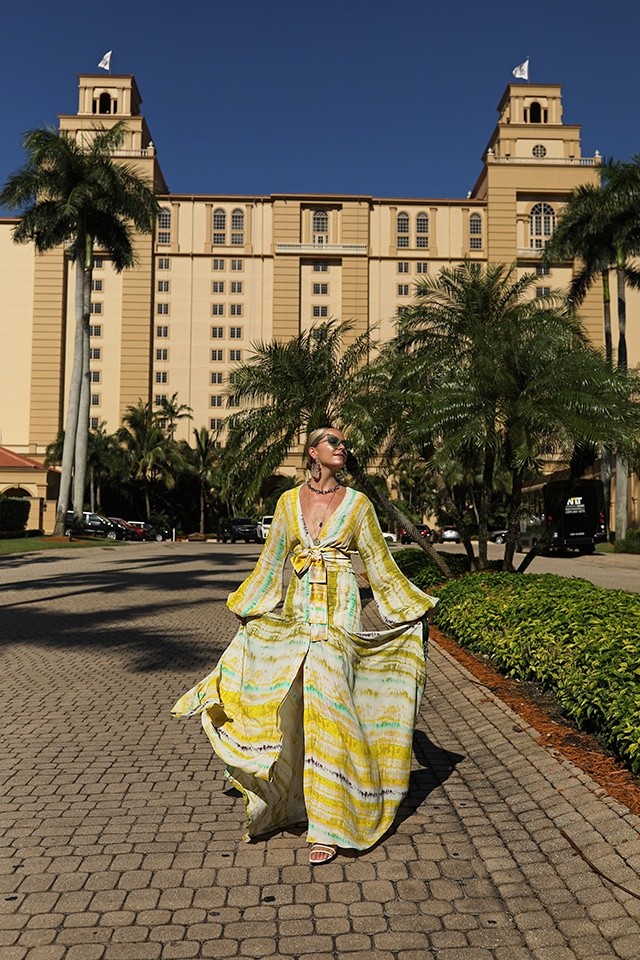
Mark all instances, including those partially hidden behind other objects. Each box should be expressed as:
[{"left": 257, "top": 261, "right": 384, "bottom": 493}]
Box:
[{"left": 309, "top": 843, "right": 338, "bottom": 865}]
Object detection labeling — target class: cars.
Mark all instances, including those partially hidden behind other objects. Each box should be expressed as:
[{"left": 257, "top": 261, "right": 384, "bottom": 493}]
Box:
[
  {"left": 63, "top": 511, "right": 170, "bottom": 541},
  {"left": 257, "top": 515, "right": 274, "bottom": 543},
  {"left": 221, "top": 517, "right": 256, "bottom": 543},
  {"left": 437, "top": 525, "right": 460, "bottom": 543},
  {"left": 401, "top": 524, "right": 435, "bottom": 544},
  {"left": 489, "top": 529, "right": 508, "bottom": 544}
]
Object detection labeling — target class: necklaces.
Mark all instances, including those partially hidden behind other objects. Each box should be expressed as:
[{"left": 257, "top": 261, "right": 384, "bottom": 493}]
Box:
[
  {"left": 307, "top": 479, "right": 339, "bottom": 494},
  {"left": 307, "top": 486, "right": 336, "bottom": 546}
]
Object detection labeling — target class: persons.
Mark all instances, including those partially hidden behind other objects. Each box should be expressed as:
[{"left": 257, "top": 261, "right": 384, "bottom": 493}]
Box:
[{"left": 171, "top": 420, "right": 440, "bottom": 864}]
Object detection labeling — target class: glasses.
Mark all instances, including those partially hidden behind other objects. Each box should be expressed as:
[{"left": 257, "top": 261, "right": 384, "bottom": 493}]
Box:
[{"left": 313, "top": 434, "right": 352, "bottom": 448}]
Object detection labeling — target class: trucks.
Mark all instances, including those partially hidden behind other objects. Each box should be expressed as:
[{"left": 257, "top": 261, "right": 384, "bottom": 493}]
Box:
[{"left": 514, "top": 479, "right": 608, "bottom": 556}]
